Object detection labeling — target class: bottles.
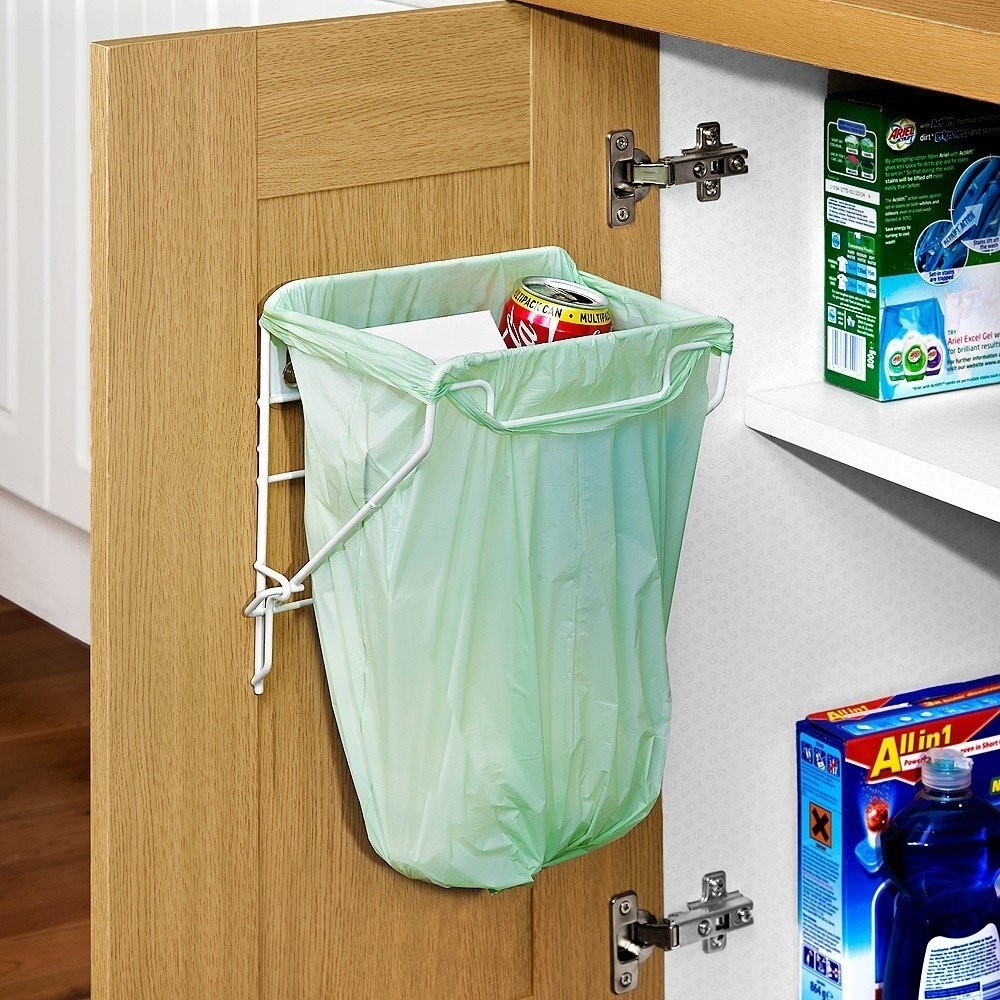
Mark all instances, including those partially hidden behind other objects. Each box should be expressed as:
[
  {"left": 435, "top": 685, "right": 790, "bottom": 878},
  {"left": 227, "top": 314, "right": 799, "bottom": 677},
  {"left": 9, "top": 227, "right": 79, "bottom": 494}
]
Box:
[{"left": 883, "top": 749, "right": 1000, "bottom": 1000}]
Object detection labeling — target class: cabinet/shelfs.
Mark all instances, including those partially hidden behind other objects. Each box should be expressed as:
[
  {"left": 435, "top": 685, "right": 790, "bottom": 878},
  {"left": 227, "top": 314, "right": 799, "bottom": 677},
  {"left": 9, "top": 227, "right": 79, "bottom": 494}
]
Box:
[{"left": 94, "top": 0, "right": 1000, "bottom": 1000}]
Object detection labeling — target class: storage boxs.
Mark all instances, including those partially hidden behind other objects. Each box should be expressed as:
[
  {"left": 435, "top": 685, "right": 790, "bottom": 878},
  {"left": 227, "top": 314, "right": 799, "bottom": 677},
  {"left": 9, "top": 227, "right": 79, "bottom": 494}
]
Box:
[
  {"left": 795, "top": 673, "right": 1000, "bottom": 1000},
  {"left": 823, "top": 70, "right": 1000, "bottom": 402}
]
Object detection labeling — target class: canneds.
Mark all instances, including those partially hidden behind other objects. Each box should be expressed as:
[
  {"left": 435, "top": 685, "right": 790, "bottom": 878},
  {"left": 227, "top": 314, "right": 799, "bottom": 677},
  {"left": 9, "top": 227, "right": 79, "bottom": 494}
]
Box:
[{"left": 497, "top": 276, "right": 613, "bottom": 347}]
